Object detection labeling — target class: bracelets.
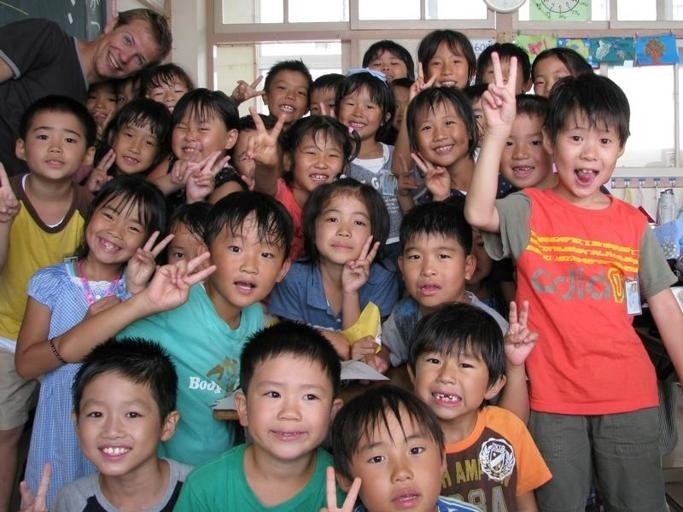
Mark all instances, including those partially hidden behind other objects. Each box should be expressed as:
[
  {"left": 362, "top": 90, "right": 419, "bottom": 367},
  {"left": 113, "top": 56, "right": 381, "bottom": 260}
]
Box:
[{"left": 49, "top": 337, "right": 68, "bottom": 365}]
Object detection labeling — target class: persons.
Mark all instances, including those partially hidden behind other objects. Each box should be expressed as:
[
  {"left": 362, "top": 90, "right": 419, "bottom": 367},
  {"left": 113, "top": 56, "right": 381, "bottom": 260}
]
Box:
[
  {"left": 144, "top": 87, "right": 250, "bottom": 206},
  {"left": 19, "top": 337, "right": 195, "bottom": 512},
  {"left": 117, "top": 70, "right": 145, "bottom": 116},
  {"left": 464, "top": 51, "right": 683, "bottom": 512},
  {"left": 318, "top": 385, "right": 484, "bottom": 512},
  {"left": 0, "top": 93, "right": 99, "bottom": 512},
  {"left": 531, "top": 47, "right": 595, "bottom": 99},
  {"left": 397, "top": 153, "right": 509, "bottom": 322},
  {"left": 494, "top": 93, "right": 610, "bottom": 304},
  {"left": 144, "top": 63, "right": 194, "bottom": 114},
  {"left": 359, "top": 200, "right": 540, "bottom": 426},
  {"left": 320, "top": 67, "right": 403, "bottom": 269},
  {"left": 230, "top": 60, "right": 313, "bottom": 133},
  {"left": 86, "top": 81, "right": 119, "bottom": 147},
  {"left": 166, "top": 200, "right": 214, "bottom": 274},
  {"left": 362, "top": 40, "right": 414, "bottom": 83},
  {"left": 59, "top": 189, "right": 350, "bottom": 466},
  {"left": 172, "top": 320, "right": 347, "bottom": 511},
  {"left": 229, "top": 113, "right": 286, "bottom": 190},
  {"left": 475, "top": 42, "right": 532, "bottom": 96},
  {"left": 390, "top": 30, "right": 478, "bottom": 176},
  {"left": 407, "top": 299, "right": 553, "bottom": 512},
  {"left": 247, "top": 105, "right": 362, "bottom": 262},
  {"left": 309, "top": 72, "right": 346, "bottom": 119},
  {"left": 0, "top": 8, "right": 173, "bottom": 178},
  {"left": 269, "top": 176, "right": 400, "bottom": 360},
  {"left": 78, "top": 96, "right": 173, "bottom": 197},
  {"left": 389, "top": 78, "right": 417, "bottom": 128},
  {"left": 13, "top": 175, "right": 176, "bottom": 512},
  {"left": 460, "top": 83, "right": 492, "bottom": 142},
  {"left": 406, "top": 86, "right": 512, "bottom": 205}
]
